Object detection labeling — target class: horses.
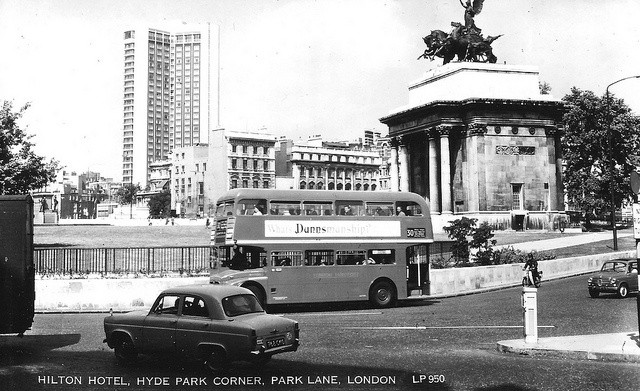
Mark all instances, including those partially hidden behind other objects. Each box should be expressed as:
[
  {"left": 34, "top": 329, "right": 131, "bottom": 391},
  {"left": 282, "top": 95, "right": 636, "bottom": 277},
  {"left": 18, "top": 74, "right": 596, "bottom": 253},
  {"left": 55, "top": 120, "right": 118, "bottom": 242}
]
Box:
[{"left": 417, "top": 28, "right": 503, "bottom": 64}]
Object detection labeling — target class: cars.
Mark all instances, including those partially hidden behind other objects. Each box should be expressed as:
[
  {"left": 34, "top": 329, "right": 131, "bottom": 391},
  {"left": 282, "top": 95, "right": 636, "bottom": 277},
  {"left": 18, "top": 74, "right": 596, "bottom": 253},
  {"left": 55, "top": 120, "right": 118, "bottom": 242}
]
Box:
[{"left": 105, "top": 283, "right": 299, "bottom": 374}]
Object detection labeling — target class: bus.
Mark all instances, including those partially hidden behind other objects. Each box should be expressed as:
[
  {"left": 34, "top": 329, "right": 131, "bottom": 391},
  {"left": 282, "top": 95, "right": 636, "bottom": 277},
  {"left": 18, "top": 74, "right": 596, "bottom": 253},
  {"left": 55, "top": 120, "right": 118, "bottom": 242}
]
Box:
[{"left": 209, "top": 187, "right": 434, "bottom": 313}]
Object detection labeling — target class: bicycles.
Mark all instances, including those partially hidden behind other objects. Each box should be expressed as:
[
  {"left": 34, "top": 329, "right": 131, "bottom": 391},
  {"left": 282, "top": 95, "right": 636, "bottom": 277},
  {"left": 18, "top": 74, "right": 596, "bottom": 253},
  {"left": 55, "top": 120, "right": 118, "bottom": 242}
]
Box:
[{"left": 522, "top": 268, "right": 543, "bottom": 287}]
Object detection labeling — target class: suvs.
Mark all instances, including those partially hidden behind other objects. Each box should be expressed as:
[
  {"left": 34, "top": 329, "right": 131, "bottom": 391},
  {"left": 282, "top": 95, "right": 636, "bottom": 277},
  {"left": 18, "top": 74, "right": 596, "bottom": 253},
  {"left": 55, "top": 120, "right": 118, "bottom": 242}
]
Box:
[{"left": 587, "top": 258, "right": 638, "bottom": 297}]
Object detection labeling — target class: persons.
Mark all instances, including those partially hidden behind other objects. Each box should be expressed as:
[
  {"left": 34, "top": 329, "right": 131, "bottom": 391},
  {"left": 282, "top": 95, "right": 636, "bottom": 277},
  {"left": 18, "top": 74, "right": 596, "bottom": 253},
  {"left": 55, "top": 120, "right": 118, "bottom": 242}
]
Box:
[
  {"left": 396, "top": 206, "right": 406, "bottom": 217},
  {"left": 252, "top": 206, "right": 264, "bottom": 216},
  {"left": 343, "top": 206, "right": 353, "bottom": 216},
  {"left": 522, "top": 254, "right": 539, "bottom": 284}
]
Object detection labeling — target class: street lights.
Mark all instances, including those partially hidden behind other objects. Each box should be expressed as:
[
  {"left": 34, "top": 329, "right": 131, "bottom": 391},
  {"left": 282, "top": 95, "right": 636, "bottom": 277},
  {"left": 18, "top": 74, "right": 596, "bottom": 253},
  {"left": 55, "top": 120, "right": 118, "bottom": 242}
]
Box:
[{"left": 606, "top": 75, "right": 640, "bottom": 250}]
